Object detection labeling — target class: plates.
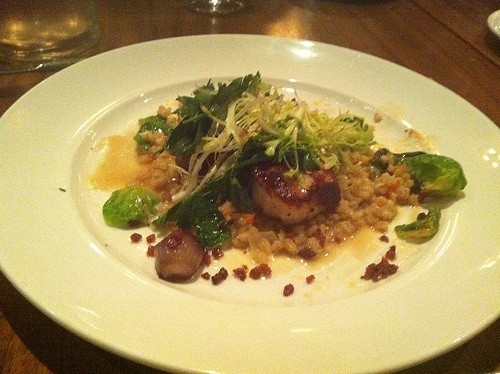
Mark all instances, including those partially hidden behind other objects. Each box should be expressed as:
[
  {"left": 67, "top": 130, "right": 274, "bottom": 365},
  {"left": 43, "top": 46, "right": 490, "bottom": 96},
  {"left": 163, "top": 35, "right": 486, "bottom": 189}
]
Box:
[{"left": 0, "top": 33, "right": 500, "bottom": 374}]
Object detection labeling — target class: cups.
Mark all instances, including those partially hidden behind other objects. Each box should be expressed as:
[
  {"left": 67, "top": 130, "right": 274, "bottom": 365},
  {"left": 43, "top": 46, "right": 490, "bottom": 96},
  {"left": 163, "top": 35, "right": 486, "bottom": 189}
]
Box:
[
  {"left": 185, "top": 0, "right": 249, "bottom": 15},
  {"left": 1, "top": 0, "right": 99, "bottom": 75}
]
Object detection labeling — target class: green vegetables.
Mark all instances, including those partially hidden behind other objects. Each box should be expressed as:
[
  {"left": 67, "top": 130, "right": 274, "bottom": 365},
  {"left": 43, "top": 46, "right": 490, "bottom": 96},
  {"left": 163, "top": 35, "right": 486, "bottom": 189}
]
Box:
[{"left": 103, "top": 70, "right": 468, "bottom": 281}]
